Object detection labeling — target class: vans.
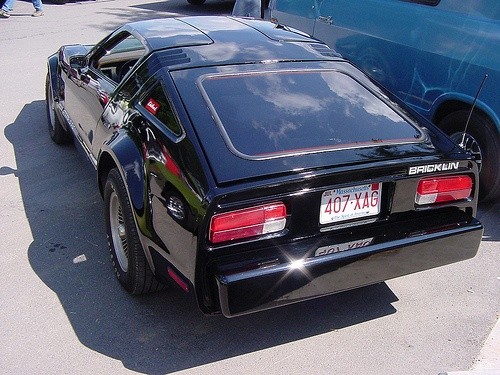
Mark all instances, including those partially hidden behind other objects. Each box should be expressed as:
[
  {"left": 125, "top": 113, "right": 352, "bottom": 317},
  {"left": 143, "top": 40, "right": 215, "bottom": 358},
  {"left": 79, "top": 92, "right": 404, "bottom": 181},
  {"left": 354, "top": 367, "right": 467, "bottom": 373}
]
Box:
[{"left": 232, "top": 0, "right": 500, "bottom": 211}]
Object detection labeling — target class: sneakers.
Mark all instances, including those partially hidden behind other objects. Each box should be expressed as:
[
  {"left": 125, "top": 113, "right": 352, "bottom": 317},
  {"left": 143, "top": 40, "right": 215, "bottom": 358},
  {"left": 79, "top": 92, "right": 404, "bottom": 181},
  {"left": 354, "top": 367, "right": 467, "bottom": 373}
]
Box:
[
  {"left": 31, "top": 10, "right": 45, "bottom": 17},
  {"left": 0, "top": 8, "right": 10, "bottom": 17}
]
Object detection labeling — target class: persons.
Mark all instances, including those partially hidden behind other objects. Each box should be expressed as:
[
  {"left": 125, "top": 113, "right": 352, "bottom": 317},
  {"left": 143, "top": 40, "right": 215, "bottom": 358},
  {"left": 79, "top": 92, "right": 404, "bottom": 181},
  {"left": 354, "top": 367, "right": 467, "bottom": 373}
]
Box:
[{"left": 0, "top": 0, "right": 45, "bottom": 17}]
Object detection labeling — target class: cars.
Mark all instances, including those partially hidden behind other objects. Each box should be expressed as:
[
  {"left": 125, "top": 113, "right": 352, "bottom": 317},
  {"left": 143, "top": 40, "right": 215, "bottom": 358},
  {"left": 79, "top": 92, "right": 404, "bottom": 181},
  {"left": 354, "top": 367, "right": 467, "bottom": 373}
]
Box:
[{"left": 46, "top": 15, "right": 484, "bottom": 317}]
